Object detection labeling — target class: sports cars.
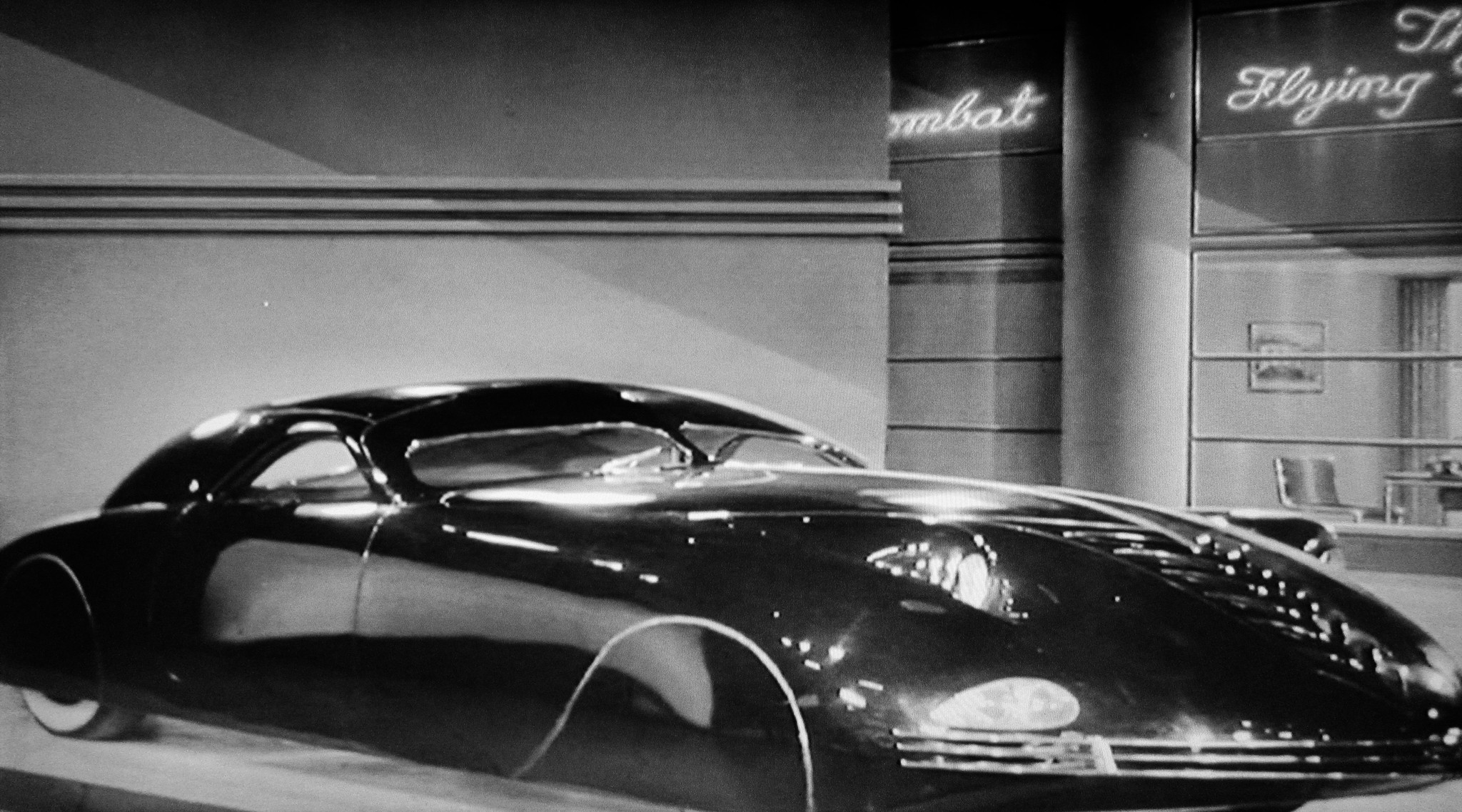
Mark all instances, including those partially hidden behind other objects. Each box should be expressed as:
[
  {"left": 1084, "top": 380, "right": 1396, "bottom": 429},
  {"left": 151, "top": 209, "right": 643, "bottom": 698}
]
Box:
[{"left": 0, "top": 381, "right": 1462, "bottom": 812}]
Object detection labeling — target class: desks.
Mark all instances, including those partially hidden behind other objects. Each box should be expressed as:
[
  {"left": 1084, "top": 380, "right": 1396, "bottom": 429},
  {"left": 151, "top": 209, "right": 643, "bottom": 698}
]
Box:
[{"left": 1379, "top": 466, "right": 1462, "bottom": 524}]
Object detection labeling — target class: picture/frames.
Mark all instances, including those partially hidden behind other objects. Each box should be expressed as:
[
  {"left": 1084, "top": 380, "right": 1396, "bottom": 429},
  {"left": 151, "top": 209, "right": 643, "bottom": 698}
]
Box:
[{"left": 1247, "top": 317, "right": 1327, "bottom": 395}]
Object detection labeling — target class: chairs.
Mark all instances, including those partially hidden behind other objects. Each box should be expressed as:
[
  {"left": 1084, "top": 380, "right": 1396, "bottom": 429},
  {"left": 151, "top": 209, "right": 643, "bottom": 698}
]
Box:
[{"left": 1272, "top": 456, "right": 1403, "bottom": 524}]
